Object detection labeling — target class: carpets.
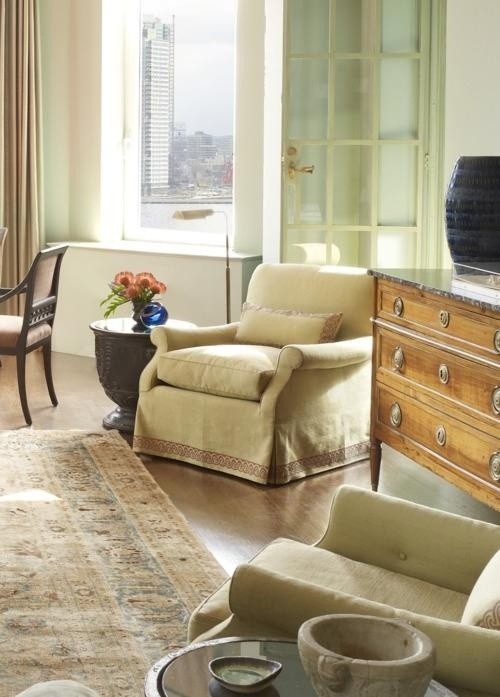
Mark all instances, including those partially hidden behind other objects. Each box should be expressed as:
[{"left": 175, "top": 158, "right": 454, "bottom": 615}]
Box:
[{"left": 0, "top": 430, "right": 230, "bottom": 696}]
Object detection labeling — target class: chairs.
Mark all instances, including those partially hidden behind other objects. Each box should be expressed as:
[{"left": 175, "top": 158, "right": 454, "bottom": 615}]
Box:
[
  {"left": 186, "top": 484, "right": 500, "bottom": 697},
  {"left": 0, "top": 244, "right": 71, "bottom": 425},
  {"left": 138, "top": 263, "right": 372, "bottom": 487}
]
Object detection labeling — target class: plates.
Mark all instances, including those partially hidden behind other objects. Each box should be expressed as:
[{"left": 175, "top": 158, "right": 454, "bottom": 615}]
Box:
[{"left": 207, "top": 655, "right": 283, "bottom": 693}]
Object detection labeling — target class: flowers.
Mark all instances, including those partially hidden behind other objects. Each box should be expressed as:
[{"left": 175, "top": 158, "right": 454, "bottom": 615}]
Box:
[{"left": 100, "top": 270, "right": 167, "bottom": 320}]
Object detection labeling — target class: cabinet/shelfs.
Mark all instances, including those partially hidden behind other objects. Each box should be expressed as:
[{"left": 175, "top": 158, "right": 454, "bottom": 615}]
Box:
[{"left": 369, "top": 276, "right": 500, "bottom": 514}]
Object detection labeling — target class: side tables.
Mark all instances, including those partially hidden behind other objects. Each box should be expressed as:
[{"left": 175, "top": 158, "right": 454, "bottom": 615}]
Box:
[
  {"left": 143, "top": 638, "right": 461, "bottom": 697},
  {"left": 88, "top": 318, "right": 197, "bottom": 433}
]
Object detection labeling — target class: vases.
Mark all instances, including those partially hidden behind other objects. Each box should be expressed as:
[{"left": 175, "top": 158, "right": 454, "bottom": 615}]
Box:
[{"left": 133, "top": 304, "right": 147, "bottom": 322}]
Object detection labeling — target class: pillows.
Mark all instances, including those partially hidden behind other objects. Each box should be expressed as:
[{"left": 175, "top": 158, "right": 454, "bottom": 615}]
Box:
[
  {"left": 234, "top": 301, "right": 344, "bottom": 348},
  {"left": 461, "top": 549, "right": 500, "bottom": 631}
]
